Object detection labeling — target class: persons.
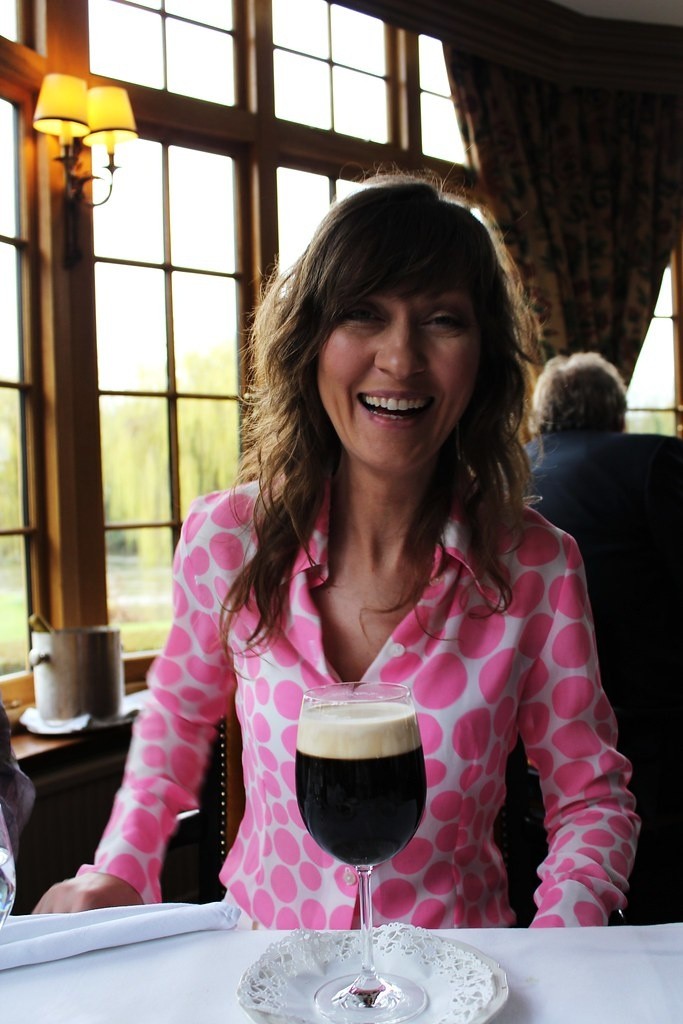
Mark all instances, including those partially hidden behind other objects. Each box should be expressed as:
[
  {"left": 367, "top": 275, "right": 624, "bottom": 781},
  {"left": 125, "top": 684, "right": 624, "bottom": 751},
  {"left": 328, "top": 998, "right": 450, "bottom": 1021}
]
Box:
[
  {"left": 522, "top": 351, "right": 683, "bottom": 926},
  {"left": 31, "top": 181, "right": 641, "bottom": 932}
]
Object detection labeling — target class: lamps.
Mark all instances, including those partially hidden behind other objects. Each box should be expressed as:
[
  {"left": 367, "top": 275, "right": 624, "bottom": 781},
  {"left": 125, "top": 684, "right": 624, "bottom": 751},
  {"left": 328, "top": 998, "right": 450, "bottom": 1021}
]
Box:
[{"left": 32, "top": 73, "right": 140, "bottom": 275}]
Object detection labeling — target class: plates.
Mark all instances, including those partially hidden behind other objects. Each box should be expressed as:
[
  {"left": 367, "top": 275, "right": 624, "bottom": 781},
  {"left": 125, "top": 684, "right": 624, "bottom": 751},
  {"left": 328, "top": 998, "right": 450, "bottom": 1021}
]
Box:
[{"left": 237, "top": 929, "right": 510, "bottom": 1024}]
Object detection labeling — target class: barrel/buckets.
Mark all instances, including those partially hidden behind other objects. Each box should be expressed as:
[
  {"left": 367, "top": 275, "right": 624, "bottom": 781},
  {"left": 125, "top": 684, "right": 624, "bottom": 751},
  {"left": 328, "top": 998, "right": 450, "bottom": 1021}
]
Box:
[{"left": 30, "top": 625, "right": 124, "bottom": 723}]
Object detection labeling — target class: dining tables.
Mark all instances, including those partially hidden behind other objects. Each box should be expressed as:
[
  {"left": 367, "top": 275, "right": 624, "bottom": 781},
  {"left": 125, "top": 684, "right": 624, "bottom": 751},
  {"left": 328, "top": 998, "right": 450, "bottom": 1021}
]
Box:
[{"left": 0, "top": 922, "right": 682, "bottom": 1023}]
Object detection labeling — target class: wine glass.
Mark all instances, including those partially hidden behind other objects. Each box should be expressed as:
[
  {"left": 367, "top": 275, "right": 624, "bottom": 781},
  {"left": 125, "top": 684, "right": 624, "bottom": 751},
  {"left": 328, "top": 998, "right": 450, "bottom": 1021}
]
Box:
[{"left": 295, "top": 681, "right": 430, "bottom": 1024}]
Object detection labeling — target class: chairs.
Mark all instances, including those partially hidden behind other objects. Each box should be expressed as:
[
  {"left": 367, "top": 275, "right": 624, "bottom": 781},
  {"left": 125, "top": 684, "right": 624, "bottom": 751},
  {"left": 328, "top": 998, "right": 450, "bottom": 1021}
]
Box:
[{"left": 218, "top": 711, "right": 531, "bottom": 929}]
